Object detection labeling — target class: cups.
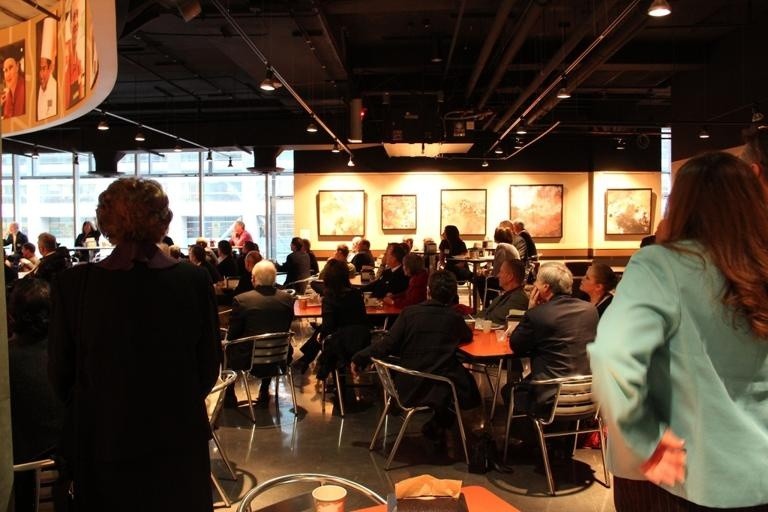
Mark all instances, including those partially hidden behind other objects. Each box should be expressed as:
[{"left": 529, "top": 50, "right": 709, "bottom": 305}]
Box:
[
  {"left": 482, "top": 321, "right": 492, "bottom": 333},
  {"left": 368, "top": 298, "right": 377, "bottom": 305},
  {"left": 496, "top": 330, "right": 504, "bottom": 342},
  {"left": 300, "top": 297, "right": 307, "bottom": 309},
  {"left": 312, "top": 485, "right": 348, "bottom": 512}
]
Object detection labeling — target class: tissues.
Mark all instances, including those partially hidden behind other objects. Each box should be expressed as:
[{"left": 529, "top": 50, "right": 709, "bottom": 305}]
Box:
[{"left": 386, "top": 474, "right": 468, "bottom": 512}]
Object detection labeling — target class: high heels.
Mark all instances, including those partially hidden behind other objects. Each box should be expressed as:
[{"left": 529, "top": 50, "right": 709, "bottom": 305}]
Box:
[{"left": 292, "top": 360, "right": 309, "bottom": 374}]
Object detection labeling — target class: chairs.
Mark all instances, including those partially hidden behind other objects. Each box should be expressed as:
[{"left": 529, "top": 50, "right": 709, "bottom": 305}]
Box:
[
  {"left": 225, "top": 331, "right": 299, "bottom": 421},
  {"left": 204, "top": 371, "right": 236, "bottom": 506},
  {"left": 367, "top": 356, "right": 471, "bottom": 471},
  {"left": 209, "top": 244, "right": 610, "bottom": 363},
  {"left": 489, "top": 375, "right": 610, "bottom": 497},
  {"left": 238, "top": 473, "right": 384, "bottom": 511}
]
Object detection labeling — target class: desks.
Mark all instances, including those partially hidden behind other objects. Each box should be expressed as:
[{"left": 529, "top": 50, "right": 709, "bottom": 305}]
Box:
[
  {"left": 355, "top": 485, "right": 518, "bottom": 511},
  {"left": 461, "top": 327, "right": 514, "bottom": 429}
]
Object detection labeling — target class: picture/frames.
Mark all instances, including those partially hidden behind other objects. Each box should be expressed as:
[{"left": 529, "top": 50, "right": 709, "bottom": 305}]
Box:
[
  {"left": 440, "top": 188, "right": 487, "bottom": 241},
  {"left": 315, "top": 189, "right": 368, "bottom": 243},
  {"left": 381, "top": 193, "right": 418, "bottom": 235},
  {"left": 508, "top": 183, "right": 564, "bottom": 243},
  {"left": 603, "top": 188, "right": 657, "bottom": 240}
]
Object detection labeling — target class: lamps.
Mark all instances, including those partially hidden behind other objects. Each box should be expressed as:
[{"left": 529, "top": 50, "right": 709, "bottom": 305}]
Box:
[
  {"left": 261, "top": 64, "right": 357, "bottom": 168},
  {"left": 91, "top": 106, "right": 239, "bottom": 170},
  {"left": 477, "top": 1, "right": 672, "bottom": 168}
]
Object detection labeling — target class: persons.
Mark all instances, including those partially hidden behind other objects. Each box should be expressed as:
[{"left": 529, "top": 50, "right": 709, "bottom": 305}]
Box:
[
  {"left": 3, "top": 219, "right": 102, "bottom": 289},
  {"left": 292, "top": 221, "right": 538, "bottom": 412},
  {"left": 740, "top": 126, "right": 768, "bottom": 193},
  {"left": 225, "top": 259, "right": 294, "bottom": 409},
  {"left": 5, "top": 277, "right": 53, "bottom": 417},
  {"left": 49, "top": 176, "right": 223, "bottom": 512},
  {"left": 507, "top": 259, "right": 599, "bottom": 470},
  {"left": 0, "top": 0, "right": 82, "bottom": 121},
  {"left": 352, "top": 270, "right": 480, "bottom": 446},
  {"left": 580, "top": 260, "right": 617, "bottom": 317},
  {"left": 160, "top": 220, "right": 319, "bottom": 307},
  {"left": 592, "top": 152, "right": 768, "bottom": 512}
]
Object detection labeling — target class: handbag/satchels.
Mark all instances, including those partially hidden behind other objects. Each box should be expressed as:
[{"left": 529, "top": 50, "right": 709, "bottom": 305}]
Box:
[{"left": 456, "top": 431, "right": 513, "bottom": 474}]
